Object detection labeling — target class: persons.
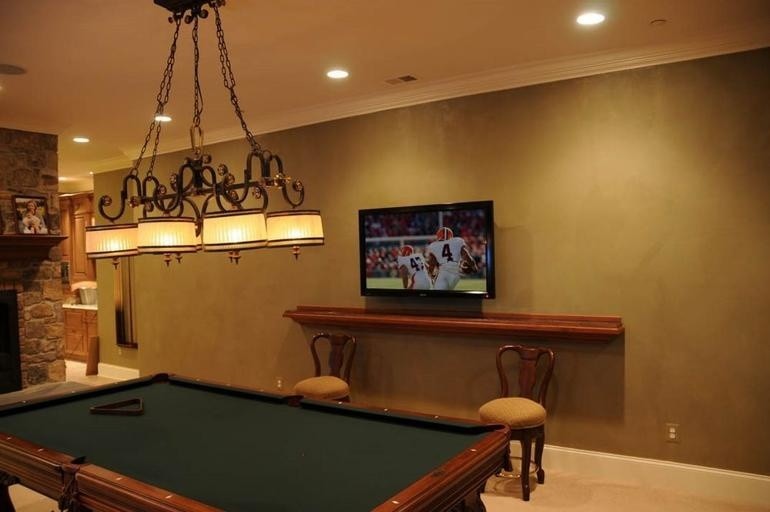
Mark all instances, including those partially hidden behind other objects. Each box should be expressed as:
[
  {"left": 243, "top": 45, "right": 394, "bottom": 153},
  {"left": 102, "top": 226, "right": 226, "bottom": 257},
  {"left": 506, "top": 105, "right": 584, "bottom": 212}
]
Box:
[
  {"left": 21, "top": 199, "right": 45, "bottom": 234},
  {"left": 22, "top": 211, "right": 40, "bottom": 235},
  {"left": 428, "top": 226, "right": 480, "bottom": 291},
  {"left": 397, "top": 245, "right": 431, "bottom": 290},
  {"left": 364, "top": 211, "right": 486, "bottom": 279}
]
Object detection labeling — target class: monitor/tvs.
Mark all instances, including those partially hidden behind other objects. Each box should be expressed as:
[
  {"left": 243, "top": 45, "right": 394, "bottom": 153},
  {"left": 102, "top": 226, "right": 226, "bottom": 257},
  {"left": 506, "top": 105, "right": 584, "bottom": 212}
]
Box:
[{"left": 358, "top": 198, "right": 497, "bottom": 299}]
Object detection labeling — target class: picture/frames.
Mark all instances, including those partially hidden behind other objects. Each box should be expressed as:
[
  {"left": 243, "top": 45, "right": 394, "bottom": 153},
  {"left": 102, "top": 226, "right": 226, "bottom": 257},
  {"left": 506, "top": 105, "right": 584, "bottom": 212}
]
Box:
[{"left": 11, "top": 193, "right": 52, "bottom": 235}]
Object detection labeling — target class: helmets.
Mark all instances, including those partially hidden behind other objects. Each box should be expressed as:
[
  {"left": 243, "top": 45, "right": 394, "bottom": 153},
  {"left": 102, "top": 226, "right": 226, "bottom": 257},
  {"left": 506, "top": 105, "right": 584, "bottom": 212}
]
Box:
[
  {"left": 400, "top": 244, "right": 412, "bottom": 257},
  {"left": 435, "top": 227, "right": 454, "bottom": 241}
]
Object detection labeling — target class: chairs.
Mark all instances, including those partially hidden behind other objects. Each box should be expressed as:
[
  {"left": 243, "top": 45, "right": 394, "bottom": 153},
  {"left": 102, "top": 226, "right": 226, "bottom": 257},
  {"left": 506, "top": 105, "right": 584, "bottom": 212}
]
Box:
[
  {"left": 479, "top": 344, "right": 556, "bottom": 501},
  {"left": 294, "top": 331, "right": 356, "bottom": 406}
]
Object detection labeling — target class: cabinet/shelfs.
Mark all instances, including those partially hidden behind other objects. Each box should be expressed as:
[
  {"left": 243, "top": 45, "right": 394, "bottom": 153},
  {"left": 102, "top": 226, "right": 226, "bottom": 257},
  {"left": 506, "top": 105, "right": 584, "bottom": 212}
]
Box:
[
  {"left": 58, "top": 193, "right": 96, "bottom": 282},
  {"left": 60, "top": 305, "right": 99, "bottom": 364}
]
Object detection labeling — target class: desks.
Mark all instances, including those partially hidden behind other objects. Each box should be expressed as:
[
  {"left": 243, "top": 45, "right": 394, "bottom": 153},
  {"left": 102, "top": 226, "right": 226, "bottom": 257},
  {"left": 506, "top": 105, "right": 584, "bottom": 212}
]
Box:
[{"left": 0, "top": 369, "right": 511, "bottom": 511}]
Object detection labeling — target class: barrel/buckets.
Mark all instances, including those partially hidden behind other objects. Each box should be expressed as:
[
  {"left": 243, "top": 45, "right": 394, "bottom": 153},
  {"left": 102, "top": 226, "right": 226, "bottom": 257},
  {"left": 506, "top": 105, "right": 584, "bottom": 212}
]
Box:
[{"left": 80, "top": 288, "right": 97, "bottom": 304}]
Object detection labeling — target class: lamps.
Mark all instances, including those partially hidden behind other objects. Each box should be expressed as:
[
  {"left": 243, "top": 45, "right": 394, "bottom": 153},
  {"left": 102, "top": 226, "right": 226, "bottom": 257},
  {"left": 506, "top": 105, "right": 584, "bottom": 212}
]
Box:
[{"left": 84, "top": 0, "right": 325, "bottom": 271}]
